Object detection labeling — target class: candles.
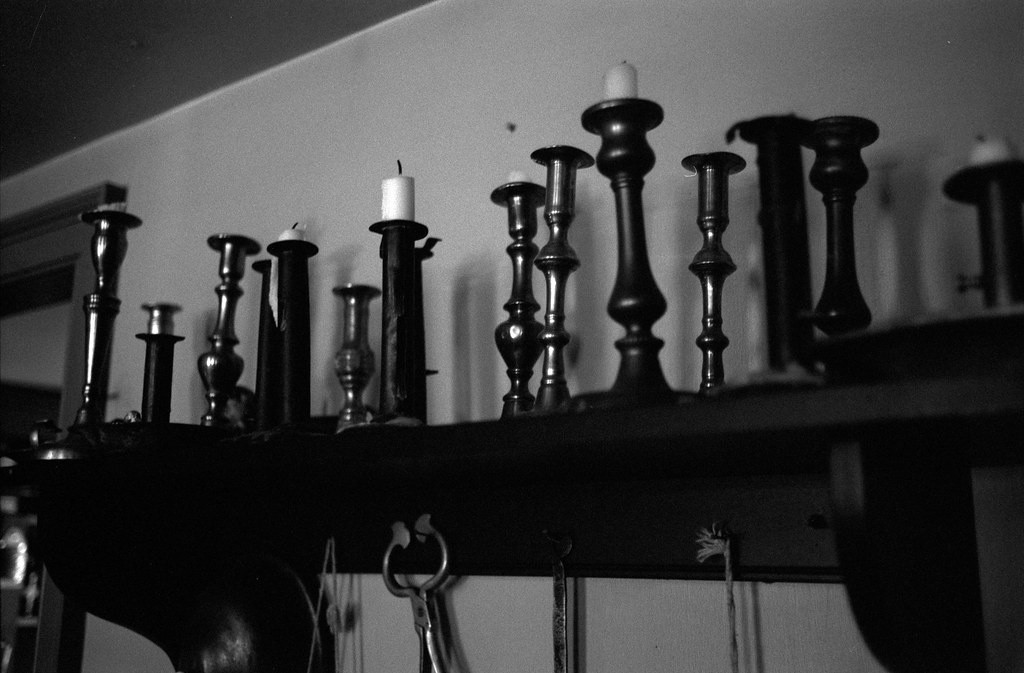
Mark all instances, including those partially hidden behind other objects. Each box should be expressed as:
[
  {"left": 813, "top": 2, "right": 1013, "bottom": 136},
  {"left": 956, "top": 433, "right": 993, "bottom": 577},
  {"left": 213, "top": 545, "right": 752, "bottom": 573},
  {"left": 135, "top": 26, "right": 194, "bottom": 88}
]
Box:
[
  {"left": 603, "top": 60, "right": 638, "bottom": 100},
  {"left": 965, "top": 136, "right": 1008, "bottom": 164},
  {"left": 380, "top": 161, "right": 415, "bottom": 222},
  {"left": 276, "top": 222, "right": 304, "bottom": 242}
]
